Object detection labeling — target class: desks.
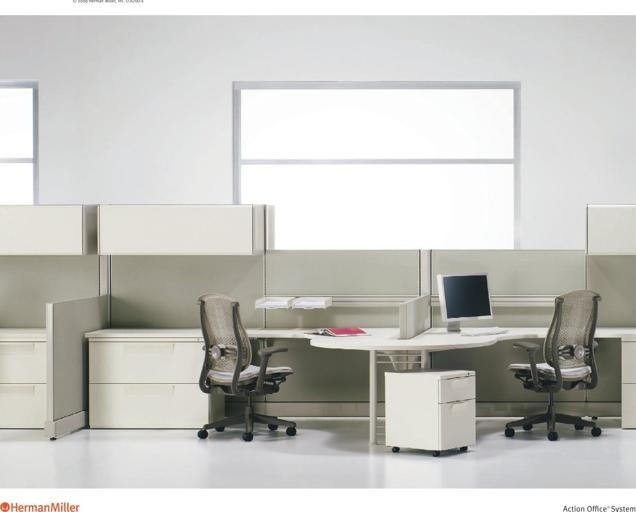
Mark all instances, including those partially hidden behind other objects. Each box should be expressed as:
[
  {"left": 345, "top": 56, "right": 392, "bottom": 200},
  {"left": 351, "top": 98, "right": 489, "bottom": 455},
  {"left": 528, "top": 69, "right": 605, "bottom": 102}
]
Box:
[{"left": 85, "top": 326, "right": 636, "bottom": 448}]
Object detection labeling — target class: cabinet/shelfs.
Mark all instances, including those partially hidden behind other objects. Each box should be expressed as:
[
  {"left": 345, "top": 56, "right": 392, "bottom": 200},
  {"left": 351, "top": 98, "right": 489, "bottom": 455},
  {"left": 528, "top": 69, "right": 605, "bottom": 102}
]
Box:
[
  {"left": 586, "top": 203, "right": 636, "bottom": 256},
  {"left": 384, "top": 367, "right": 475, "bottom": 457},
  {"left": 96, "top": 204, "right": 263, "bottom": 256},
  {"left": 0, "top": 204, "right": 96, "bottom": 257},
  {"left": 0, "top": 333, "right": 88, "bottom": 442}
]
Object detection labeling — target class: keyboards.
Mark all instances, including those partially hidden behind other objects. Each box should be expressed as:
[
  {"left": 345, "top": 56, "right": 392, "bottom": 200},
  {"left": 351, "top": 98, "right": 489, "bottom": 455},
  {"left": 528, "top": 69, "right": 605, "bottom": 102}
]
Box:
[{"left": 462, "top": 326, "right": 509, "bottom": 335}]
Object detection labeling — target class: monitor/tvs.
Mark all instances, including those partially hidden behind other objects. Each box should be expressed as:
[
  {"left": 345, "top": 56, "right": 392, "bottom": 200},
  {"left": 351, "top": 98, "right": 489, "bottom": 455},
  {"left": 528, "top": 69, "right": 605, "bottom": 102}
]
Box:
[{"left": 437, "top": 272, "right": 493, "bottom": 332}]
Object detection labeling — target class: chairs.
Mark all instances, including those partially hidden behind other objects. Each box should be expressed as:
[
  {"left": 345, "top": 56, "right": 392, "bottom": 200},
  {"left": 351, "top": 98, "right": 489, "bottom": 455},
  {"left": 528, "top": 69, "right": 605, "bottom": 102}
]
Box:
[
  {"left": 196, "top": 292, "right": 297, "bottom": 440},
  {"left": 504, "top": 289, "right": 602, "bottom": 439}
]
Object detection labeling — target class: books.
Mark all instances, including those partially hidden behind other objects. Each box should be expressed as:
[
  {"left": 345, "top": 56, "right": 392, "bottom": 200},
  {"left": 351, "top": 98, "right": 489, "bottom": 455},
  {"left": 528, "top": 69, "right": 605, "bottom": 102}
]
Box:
[{"left": 306, "top": 325, "right": 371, "bottom": 337}]
